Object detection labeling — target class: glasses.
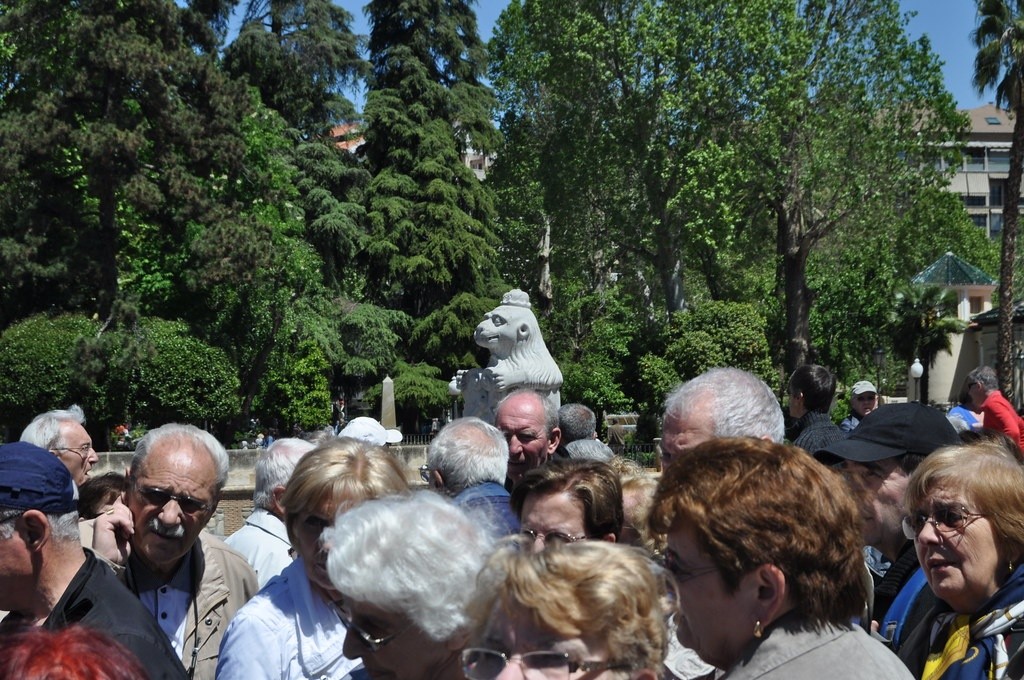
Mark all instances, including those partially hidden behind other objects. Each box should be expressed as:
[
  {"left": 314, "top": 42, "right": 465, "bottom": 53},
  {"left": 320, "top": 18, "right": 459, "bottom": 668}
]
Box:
[
  {"left": 856, "top": 397, "right": 874, "bottom": 401},
  {"left": 419, "top": 464, "right": 430, "bottom": 482},
  {"left": 140, "top": 489, "right": 211, "bottom": 516},
  {"left": 52, "top": 446, "right": 90, "bottom": 455},
  {"left": 651, "top": 548, "right": 721, "bottom": 581},
  {"left": 329, "top": 598, "right": 416, "bottom": 650},
  {"left": 902, "top": 508, "right": 985, "bottom": 540},
  {"left": 968, "top": 381, "right": 983, "bottom": 389},
  {"left": 509, "top": 527, "right": 589, "bottom": 550},
  {"left": 460, "top": 648, "right": 632, "bottom": 680}
]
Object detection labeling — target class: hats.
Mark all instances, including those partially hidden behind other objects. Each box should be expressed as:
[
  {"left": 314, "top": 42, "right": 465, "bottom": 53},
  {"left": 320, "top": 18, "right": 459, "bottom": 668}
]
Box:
[
  {"left": 338, "top": 416, "right": 403, "bottom": 446},
  {"left": 852, "top": 380, "right": 877, "bottom": 394},
  {"left": 0, "top": 442, "right": 80, "bottom": 512},
  {"left": 813, "top": 404, "right": 960, "bottom": 466}
]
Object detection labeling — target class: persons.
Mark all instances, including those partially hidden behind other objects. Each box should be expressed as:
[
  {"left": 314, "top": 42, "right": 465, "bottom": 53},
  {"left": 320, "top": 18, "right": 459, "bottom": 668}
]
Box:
[{"left": 0, "top": 358, "right": 1024, "bottom": 680}]
